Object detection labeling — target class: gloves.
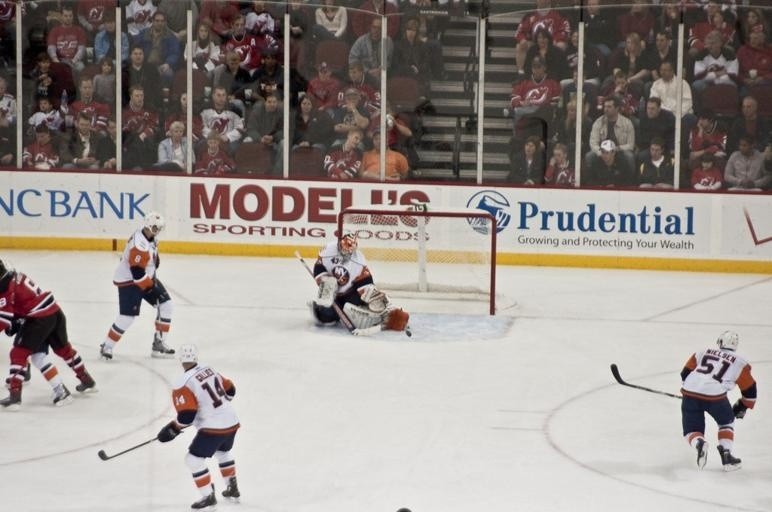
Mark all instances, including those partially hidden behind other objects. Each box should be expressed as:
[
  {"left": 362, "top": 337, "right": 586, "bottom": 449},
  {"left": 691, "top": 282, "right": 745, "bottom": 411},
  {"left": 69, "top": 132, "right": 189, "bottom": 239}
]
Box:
[
  {"left": 157, "top": 421, "right": 184, "bottom": 443},
  {"left": 732, "top": 399, "right": 747, "bottom": 418}
]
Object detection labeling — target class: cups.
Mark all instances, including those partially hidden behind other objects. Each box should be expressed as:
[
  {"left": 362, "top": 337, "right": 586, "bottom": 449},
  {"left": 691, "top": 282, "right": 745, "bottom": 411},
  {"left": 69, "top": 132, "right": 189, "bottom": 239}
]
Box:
[
  {"left": 86, "top": 46, "right": 94, "bottom": 63},
  {"left": 749, "top": 69, "right": 758, "bottom": 80},
  {"left": 163, "top": 87, "right": 169, "bottom": 102},
  {"left": 244, "top": 88, "right": 252, "bottom": 104},
  {"left": 204, "top": 86, "right": 211, "bottom": 102},
  {"left": 65, "top": 115, "right": 73, "bottom": 127}
]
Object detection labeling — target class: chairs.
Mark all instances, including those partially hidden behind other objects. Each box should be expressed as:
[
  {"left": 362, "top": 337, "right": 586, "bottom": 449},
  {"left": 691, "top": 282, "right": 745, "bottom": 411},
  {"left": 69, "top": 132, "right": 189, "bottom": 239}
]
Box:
[
  {"left": 48, "top": 39, "right": 420, "bottom": 177},
  {"left": 701, "top": 83, "right": 772, "bottom": 119}
]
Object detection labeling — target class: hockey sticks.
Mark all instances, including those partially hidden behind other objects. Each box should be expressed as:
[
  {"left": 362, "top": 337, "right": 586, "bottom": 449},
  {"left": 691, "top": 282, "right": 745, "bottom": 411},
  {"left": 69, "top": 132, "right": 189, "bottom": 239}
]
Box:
[
  {"left": 296, "top": 253, "right": 385, "bottom": 338},
  {"left": 152, "top": 270, "right": 165, "bottom": 346},
  {"left": 98, "top": 435, "right": 161, "bottom": 461},
  {"left": 612, "top": 366, "right": 682, "bottom": 398}
]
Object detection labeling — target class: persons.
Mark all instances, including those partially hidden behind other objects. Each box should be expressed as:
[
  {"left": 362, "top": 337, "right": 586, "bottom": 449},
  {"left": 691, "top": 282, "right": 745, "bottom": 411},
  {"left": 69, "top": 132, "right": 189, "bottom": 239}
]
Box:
[
  {"left": 100, "top": 213, "right": 174, "bottom": 358},
  {"left": 680, "top": 331, "right": 757, "bottom": 466},
  {"left": 0, "top": 1, "right": 472, "bottom": 182},
  {"left": 6, "top": 315, "right": 70, "bottom": 404},
  {"left": 157, "top": 345, "right": 245, "bottom": 509},
  {"left": 504, "top": 1, "right": 771, "bottom": 193},
  {"left": 312, "top": 233, "right": 409, "bottom": 331},
  {"left": 0, "top": 261, "right": 95, "bottom": 406}
]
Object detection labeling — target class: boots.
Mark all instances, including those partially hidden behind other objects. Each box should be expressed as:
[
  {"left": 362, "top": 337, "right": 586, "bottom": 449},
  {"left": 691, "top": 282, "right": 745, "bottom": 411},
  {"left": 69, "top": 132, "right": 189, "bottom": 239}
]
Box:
[
  {"left": 152, "top": 332, "right": 175, "bottom": 353},
  {"left": 53, "top": 383, "right": 71, "bottom": 403},
  {"left": 191, "top": 483, "right": 217, "bottom": 509},
  {"left": 1, "top": 390, "right": 23, "bottom": 404},
  {"left": 76, "top": 369, "right": 94, "bottom": 391},
  {"left": 222, "top": 476, "right": 240, "bottom": 498},
  {"left": 100, "top": 343, "right": 115, "bottom": 360},
  {"left": 717, "top": 445, "right": 741, "bottom": 465},
  {"left": 696, "top": 438, "right": 707, "bottom": 467}
]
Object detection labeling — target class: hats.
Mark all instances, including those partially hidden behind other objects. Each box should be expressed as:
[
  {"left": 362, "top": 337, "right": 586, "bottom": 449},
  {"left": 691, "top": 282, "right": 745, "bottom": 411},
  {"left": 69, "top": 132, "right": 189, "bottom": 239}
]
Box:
[
  {"left": 317, "top": 61, "right": 331, "bottom": 72},
  {"left": 597, "top": 140, "right": 617, "bottom": 153}
]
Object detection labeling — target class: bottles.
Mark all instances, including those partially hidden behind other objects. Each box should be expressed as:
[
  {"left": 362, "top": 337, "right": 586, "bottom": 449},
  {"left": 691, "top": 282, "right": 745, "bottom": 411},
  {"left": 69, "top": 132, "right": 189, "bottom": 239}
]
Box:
[{"left": 639, "top": 96, "right": 646, "bottom": 118}]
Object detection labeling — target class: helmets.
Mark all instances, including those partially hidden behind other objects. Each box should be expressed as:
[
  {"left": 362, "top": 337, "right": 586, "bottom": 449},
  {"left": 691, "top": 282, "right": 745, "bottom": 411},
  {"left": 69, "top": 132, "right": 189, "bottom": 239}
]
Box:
[
  {"left": 336, "top": 233, "right": 356, "bottom": 260},
  {"left": 718, "top": 330, "right": 739, "bottom": 350},
  {"left": 179, "top": 341, "right": 199, "bottom": 366},
  {"left": 144, "top": 211, "right": 167, "bottom": 236}
]
